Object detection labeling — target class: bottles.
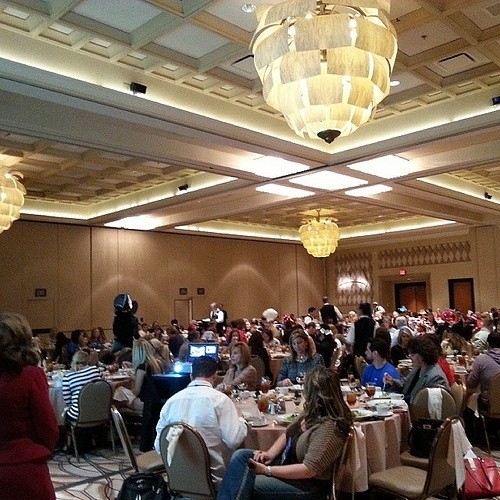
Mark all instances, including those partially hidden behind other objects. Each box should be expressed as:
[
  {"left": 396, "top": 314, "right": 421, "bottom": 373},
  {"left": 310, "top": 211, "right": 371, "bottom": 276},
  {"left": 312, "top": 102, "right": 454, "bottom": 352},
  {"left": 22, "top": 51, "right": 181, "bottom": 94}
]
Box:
[{"left": 277, "top": 397, "right": 285, "bottom": 413}]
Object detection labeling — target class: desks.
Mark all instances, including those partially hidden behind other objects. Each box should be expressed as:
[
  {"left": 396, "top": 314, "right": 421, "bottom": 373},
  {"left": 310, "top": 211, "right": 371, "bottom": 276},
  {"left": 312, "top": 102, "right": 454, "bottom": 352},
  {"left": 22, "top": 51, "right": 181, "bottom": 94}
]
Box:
[
  {"left": 399, "top": 354, "right": 480, "bottom": 419},
  {"left": 44, "top": 365, "right": 131, "bottom": 425}
]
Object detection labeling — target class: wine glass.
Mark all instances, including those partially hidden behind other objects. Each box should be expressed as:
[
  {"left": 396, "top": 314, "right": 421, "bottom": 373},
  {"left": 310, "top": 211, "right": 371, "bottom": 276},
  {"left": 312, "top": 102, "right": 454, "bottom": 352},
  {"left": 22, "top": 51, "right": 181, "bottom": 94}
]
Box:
[{"left": 296, "top": 373, "right": 305, "bottom": 395}]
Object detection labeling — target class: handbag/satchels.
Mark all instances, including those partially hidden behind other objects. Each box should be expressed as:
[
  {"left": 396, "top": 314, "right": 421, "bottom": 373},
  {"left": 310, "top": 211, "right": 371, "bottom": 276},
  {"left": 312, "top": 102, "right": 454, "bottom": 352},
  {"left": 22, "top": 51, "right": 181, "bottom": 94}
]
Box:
[
  {"left": 462, "top": 454, "right": 500, "bottom": 499},
  {"left": 408, "top": 419, "right": 445, "bottom": 459},
  {"left": 114, "top": 472, "right": 172, "bottom": 500}
]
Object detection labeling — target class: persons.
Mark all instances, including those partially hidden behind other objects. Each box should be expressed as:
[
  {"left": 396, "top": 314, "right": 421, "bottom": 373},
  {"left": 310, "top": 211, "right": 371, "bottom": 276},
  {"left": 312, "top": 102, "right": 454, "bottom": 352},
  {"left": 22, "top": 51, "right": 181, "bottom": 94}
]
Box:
[
  {"left": 319, "top": 295, "right": 343, "bottom": 329},
  {"left": 22, "top": 307, "right": 500, "bottom": 500},
  {"left": 209, "top": 301, "right": 225, "bottom": 337},
  {"left": 154, "top": 355, "right": 249, "bottom": 496},
  {"left": 336, "top": 302, "right": 380, "bottom": 368},
  {"left": 371, "top": 302, "right": 386, "bottom": 324},
  {"left": 219, "top": 303, "right": 228, "bottom": 335},
  {"left": 110, "top": 293, "right": 142, "bottom": 357},
  {"left": 215, "top": 368, "right": 357, "bottom": 500},
  {"left": 0, "top": 310, "right": 62, "bottom": 500}
]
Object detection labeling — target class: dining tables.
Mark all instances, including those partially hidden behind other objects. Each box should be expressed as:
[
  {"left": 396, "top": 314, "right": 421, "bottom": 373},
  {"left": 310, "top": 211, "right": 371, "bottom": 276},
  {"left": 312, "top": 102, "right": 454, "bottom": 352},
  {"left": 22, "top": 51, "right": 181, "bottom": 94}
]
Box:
[{"left": 233, "top": 384, "right": 412, "bottom": 492}]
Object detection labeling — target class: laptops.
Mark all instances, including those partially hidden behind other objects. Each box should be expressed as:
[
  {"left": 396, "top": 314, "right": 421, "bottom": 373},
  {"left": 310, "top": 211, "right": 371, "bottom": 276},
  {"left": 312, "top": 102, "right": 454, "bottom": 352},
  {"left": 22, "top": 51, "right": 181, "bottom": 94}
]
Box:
[{"left": 188, "top": 344, "right": 220, "bottom": 363}]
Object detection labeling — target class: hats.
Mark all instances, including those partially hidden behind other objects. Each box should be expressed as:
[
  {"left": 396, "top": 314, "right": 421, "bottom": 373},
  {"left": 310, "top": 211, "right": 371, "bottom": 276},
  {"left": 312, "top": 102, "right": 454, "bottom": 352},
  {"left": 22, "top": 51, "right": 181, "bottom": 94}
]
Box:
[
  {"left": 112, "top": 293, "right": 139, "bottom": 317},
  {"left": 372, "top": 302, "right": 379, "bottom": 305}
]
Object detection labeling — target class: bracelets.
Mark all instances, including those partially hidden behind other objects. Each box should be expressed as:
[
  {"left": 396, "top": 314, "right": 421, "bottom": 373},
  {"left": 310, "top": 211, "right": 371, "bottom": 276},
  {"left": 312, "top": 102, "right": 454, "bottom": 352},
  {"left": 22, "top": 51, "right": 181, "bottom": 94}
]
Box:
[{"left": 266, "top": 465, "right": 273, "bottom": 478}]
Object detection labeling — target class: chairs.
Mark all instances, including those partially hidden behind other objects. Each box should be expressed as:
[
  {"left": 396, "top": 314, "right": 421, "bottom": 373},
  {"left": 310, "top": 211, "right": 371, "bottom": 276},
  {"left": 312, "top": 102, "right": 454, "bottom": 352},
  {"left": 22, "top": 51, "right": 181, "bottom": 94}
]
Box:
[{"left": 66, "top": 343, "right": 500, "bottom": 500}]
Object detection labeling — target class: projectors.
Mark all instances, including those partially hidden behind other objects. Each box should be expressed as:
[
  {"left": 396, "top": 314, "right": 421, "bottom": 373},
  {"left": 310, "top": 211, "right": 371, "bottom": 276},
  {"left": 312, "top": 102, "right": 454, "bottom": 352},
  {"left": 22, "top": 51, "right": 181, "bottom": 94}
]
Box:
[{"left": 172, "top": 362, "right": 193, "bottom": 373}]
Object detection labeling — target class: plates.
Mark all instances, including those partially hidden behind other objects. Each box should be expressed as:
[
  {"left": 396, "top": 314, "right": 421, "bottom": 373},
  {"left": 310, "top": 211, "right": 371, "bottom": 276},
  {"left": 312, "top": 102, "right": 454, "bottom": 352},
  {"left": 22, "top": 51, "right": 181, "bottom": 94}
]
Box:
[
  {"left": 243, "top": 385, "right": 403, "bottom": 426},
  {"left": 271, "top": 354, "right": 291, "bottom": 358}
]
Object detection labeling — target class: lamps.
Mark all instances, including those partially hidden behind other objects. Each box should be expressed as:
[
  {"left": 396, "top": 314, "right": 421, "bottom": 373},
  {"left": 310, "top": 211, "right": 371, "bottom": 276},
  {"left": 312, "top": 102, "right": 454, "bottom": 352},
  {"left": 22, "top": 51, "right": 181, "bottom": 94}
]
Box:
[
  {"left": 0, "top": 153, "right": 29, "bottom": 234},
  {"left": 298, "top": 210, "right": 340, "bottom": 258},
  {"left": 250, "top": 0, "right": 400, "bottom": 144}
]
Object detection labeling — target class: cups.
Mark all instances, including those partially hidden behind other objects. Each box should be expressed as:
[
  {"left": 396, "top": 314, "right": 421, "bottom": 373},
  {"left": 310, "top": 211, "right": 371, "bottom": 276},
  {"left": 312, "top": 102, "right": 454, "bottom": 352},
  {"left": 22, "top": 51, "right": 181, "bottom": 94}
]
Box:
[
  {"left": 445, "top": 354, "right": 477, "bottom": 370},
  {"left": 264, "top": 343, "right": 290, "bottom": 354},
  {"left": 45, "top": 360, "right": 129, "bottom": 375},
  {"left": 218, "top": 375, "right": 404, "bottom": 414},
  {"left": 399, "top": 359, "right": 412, "bottom": 372}
]
